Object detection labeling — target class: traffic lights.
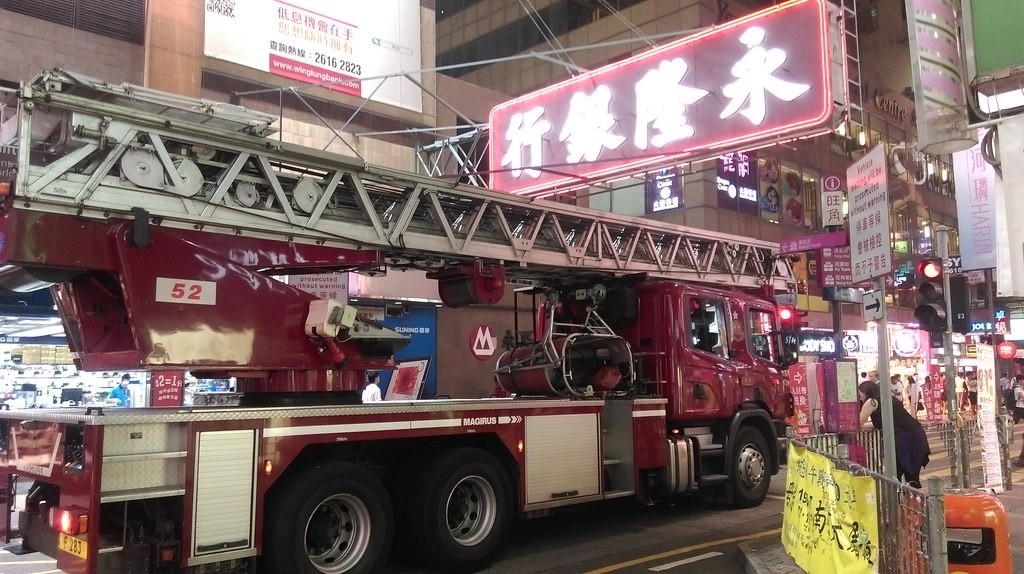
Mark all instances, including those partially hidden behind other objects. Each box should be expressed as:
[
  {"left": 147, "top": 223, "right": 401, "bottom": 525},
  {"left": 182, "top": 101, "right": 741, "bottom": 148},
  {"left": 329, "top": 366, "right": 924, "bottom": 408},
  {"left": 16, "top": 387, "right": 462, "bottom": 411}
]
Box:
[
  {"left": 914, "top": 258, "right": 949, "bottom": 334},
  {"left": 780, "top": 308, "right": 795, "bottom": 334},
  {"left": 795, "top": 310, "right": 809, "bottom": 331},
  {"left": 981, "top": 336, "right": 992, "bottom": 344}
]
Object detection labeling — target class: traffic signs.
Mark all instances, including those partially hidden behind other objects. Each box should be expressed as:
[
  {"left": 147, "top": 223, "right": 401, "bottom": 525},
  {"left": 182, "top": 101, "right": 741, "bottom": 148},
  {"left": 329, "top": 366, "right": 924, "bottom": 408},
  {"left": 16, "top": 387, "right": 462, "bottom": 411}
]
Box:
[{"left": 779, "top": 232, "right": 847, "bottom": 256}]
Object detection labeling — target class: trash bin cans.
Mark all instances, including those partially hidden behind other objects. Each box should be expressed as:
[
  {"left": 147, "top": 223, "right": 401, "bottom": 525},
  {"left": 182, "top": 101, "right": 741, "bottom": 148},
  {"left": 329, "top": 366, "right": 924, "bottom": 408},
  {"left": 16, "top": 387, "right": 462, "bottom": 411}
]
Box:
[{"left": 898, "top": 487, "right": 1013, "bottom": 574}]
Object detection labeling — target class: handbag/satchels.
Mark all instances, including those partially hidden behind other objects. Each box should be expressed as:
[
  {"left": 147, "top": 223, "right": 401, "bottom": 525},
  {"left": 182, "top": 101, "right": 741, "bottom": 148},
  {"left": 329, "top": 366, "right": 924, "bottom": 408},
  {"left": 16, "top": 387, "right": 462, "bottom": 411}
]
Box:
[{"left": 916, "top": 401, "right": 924, "bottom": 411}]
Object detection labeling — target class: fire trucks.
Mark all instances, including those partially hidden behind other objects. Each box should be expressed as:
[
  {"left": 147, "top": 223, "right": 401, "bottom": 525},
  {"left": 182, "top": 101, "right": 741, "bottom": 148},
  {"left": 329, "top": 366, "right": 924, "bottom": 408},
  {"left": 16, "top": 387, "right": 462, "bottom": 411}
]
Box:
[{"left": 0, "top": 67, "right": 798, "bottom": 574}]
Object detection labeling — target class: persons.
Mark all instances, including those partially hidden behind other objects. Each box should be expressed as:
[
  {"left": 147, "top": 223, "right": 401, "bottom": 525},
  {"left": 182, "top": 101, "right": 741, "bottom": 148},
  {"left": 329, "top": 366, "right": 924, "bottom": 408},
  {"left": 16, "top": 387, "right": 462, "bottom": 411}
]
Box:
[
  {"left": 362, "top": 372, "right": 382, "bottom": 404},
  {"left": 105, "top": 374, "right": 131, "bottom": 406},
  {"left": 858, "top": 382, "right": 932, "bottom": 503},
  {"left": 861, "top": 371, "right": 1024, "bottom": 467}
]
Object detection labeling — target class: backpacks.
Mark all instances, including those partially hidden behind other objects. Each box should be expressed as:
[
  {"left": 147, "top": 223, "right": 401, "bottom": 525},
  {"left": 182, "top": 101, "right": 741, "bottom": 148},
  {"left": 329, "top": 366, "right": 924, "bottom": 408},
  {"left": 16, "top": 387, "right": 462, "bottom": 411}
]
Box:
[{"left": 1004, "top": 386, "right": 1021, "bottom": 410}]
